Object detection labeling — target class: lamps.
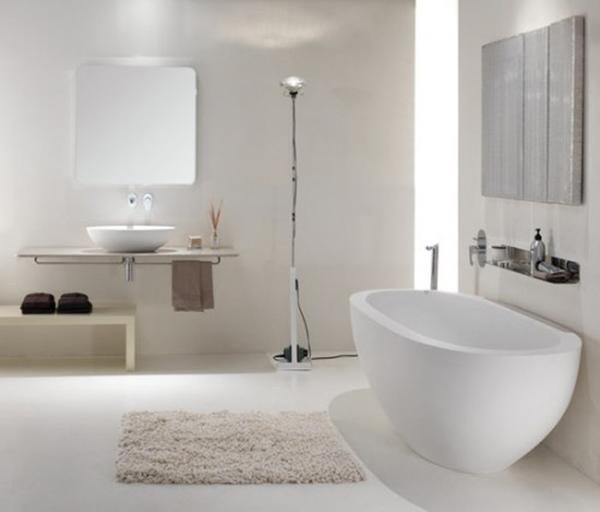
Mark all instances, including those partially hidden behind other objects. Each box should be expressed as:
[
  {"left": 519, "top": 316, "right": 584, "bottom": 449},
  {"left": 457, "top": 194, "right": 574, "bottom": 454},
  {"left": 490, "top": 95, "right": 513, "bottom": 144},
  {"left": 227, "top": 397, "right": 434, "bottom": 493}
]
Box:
[{"left": 270, "top": 74, "right": 323, "bottom": 370}]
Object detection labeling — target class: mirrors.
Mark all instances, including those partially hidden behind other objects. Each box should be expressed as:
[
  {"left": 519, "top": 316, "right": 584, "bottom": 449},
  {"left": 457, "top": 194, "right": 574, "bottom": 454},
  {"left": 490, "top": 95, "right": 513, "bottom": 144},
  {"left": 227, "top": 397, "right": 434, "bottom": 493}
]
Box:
[{"left": 70, "top": 60, "right": 202, "bottom": 195}]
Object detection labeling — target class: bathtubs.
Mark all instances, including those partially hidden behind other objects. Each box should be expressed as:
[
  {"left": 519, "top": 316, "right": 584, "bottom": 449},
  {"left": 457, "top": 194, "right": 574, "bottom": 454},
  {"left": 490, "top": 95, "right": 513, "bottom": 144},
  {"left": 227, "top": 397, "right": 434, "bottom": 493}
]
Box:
[{"left": 348, "top": 287, "right": 584, "bottom": 477}]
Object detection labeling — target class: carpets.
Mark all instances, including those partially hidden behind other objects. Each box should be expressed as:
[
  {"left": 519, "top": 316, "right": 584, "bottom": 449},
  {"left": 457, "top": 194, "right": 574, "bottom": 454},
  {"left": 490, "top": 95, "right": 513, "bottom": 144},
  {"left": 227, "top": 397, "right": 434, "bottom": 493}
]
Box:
[{"left": 112, "top": 405, "right": 371, "bottom": 491}]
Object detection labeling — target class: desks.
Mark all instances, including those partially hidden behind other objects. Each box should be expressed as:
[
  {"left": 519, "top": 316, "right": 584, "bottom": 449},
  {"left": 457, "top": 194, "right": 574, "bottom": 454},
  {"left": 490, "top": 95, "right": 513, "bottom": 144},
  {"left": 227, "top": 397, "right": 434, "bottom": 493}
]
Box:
[{"left": 0, "top": 301, "right": 142, "bottom": 371}]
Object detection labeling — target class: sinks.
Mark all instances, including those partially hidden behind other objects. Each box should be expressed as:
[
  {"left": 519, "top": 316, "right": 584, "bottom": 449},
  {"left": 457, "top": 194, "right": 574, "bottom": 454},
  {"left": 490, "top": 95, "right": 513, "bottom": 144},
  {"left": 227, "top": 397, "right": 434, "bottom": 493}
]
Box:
[{"left": 86, "top": 224, "right": 175, "bottom": 252}]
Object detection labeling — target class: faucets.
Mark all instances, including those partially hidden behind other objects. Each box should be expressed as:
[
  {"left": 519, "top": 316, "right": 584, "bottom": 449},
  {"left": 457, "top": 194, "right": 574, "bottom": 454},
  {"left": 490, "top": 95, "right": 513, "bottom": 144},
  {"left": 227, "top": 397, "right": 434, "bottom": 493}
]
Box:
[
  {"left": 423, "top": 241, "right": 441, "bottom": 291},
  {"left": 127, "top": 193, "right": 138, "bottom": 209}
]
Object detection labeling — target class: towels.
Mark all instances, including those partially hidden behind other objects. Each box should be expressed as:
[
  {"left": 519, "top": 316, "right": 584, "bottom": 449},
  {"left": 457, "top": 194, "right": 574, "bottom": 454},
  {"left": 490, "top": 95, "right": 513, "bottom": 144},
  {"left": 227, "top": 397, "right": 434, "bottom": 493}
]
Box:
[
  {"left": 21, "top": 291, "right": 93, "bottom": 317},
  {"left": 168, "top": 256, "right": 217, "bottom": 314},
  {"left": 533, "top": 269, "right": 573, "bottom": 283}
]
